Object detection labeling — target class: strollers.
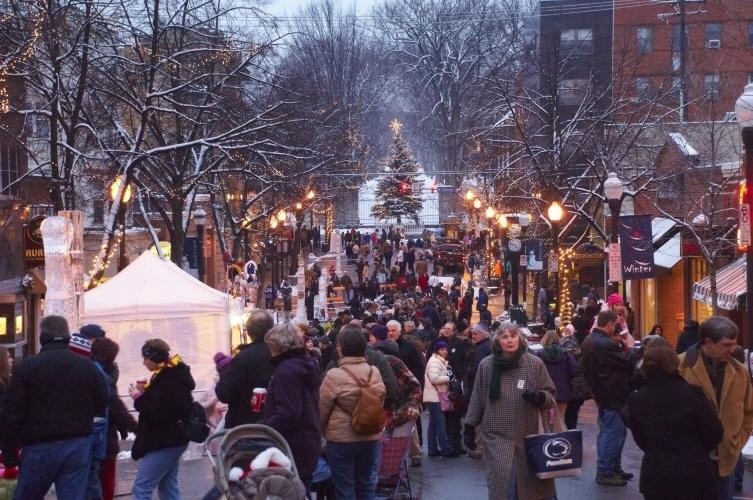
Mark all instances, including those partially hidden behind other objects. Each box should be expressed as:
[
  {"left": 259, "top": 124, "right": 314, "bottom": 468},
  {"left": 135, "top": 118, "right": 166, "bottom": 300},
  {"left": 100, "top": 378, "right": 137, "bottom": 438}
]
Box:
[
  {"left": 203, "top": 422, "right": 309, "bottom": 499},
  {"left": 379, "top": 409, "right": 414, "bottom": 500}
]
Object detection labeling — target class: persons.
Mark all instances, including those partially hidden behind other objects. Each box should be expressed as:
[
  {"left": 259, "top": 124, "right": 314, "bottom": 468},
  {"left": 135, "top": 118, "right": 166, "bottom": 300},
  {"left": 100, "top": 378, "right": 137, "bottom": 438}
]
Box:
[
  {"left": 571, "top": 281, "right": 753, "bottom": 498},
  {"left": 131, "top": 338, "right": 193, "bottom": 499},
  {"left": 205, "top": 224, "right": 583, "bottom": 499},
  {"left": 1, "top": 317, "right": 135, "bottom": 498}
]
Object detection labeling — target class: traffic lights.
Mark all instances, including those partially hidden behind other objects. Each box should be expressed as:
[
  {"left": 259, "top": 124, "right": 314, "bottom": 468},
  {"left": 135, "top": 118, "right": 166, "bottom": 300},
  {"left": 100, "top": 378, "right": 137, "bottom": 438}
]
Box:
[{"left": 399, "top": 183, "right": 407, "bottom": 193}]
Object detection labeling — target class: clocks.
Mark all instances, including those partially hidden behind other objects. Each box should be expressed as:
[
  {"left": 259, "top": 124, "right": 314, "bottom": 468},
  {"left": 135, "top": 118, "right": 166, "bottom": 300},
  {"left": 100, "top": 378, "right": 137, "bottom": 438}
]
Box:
[{"left": 508, "top": 238, "right": 521, "bottom": 253}]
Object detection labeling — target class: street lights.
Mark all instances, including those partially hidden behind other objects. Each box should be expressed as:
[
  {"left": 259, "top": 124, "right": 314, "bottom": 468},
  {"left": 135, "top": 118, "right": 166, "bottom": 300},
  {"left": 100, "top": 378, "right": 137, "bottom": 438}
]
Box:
[
  {"left": 735, "top": 82, "right": 752, "bottom": 352},
  {"left": 547, "top": 201, "right": 565, "bottom": 312},
  {"left": 193, "top": 205, "right": 207, "bottom": 283},
  {"left": 466, "top": 188, "right": 530, "bottom": 304},
  {"left": 111, "top": 175, "right": 132, "bottom": 271},
  {"left": 603, "top": 171, "right": 622, "bottom": 293},
  {"left": 243, "top": 190, "right": 315, "bottom": 308}
]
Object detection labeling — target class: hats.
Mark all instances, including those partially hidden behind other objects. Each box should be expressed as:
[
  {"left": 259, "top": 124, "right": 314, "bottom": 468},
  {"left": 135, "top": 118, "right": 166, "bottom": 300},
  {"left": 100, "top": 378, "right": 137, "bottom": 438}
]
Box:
[
  {"left": 91, "top": 339, "right": 118, "bottom": 362},
  {"left": 73, "top": 324, "right": 105, "bottom": 340},
  {"left": 608, "top": 293, "right": 623, "bottom": 307},
  {"left": 214, "top": 353, "right": 234, "bottom": 372},
  {"left": 371, "top": 326, "right": 388, "bottom": 339},
  {"left": 435, "top": 342, "right": 446, "bottom": 351}
]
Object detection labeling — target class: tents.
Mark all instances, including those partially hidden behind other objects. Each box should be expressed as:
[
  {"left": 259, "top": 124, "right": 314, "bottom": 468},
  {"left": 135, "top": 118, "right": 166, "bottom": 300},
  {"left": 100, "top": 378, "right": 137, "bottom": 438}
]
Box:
[{"left": 80, "top": 250, "right": 235, "bottom": 424}]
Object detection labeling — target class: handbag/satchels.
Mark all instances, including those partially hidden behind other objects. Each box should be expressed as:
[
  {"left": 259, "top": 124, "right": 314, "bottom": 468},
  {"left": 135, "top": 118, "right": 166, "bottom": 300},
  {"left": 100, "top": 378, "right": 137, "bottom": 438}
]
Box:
[
  {"left": 438, "top": 391, "right": 455, "bottom": 411},
  {"left": 174, "top": 402, "right": 210, "bottom": 443},
  {"left": 90, "top": 417, "right": 108, "bottom": 459},
  {"left": 525, "top": 429, "right": 583, "bottom": 479}
]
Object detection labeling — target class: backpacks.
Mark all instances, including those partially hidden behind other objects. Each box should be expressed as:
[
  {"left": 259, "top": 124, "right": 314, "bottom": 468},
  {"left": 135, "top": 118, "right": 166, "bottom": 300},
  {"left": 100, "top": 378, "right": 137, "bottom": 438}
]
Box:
[{"left": 336, "top": 365, "right": 386, "bottom": 434}]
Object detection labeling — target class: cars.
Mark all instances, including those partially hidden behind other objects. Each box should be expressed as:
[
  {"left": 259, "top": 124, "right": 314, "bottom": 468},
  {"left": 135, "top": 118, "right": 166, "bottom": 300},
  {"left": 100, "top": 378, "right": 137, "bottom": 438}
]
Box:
[{"left": 434, "top": 235, "right": 465, "bottom": 274}]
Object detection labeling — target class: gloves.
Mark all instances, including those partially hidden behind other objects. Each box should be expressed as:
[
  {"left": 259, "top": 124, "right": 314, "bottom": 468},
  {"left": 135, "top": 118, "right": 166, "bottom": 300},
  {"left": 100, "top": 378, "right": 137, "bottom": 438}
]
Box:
[
  {"left": 523, "top": 389, "right": 544, "bottom": 404},
  {"left": 465, "top": 435, "right": 478, "bottom": 450}
]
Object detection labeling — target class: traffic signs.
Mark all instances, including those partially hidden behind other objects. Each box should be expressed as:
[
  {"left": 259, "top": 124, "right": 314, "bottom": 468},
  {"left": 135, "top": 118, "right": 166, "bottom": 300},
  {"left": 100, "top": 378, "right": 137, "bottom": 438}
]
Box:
[
  {"left": 437, "top": 186, "right": 455, "bottom": 193},
  {"left": 411, "top": 183, "right": 419, "bottom": 193}
]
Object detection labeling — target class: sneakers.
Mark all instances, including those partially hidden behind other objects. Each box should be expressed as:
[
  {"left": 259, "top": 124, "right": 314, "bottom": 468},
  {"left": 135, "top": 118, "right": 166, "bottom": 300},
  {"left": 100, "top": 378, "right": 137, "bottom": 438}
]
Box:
[
  {"left": 412, "top": 458, "right": 422, "bottom": 467},
  {"left": 428, "top": 448, "right": 466, "bottom": 458},
  {"left": 597, "top": 466, "right": 633, "bottom": 486}
]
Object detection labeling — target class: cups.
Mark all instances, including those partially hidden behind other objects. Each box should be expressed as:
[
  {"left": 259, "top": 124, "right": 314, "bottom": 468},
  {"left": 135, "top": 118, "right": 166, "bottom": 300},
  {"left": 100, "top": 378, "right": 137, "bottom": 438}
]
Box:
[
  {"left": 136, "top": 379, "right": 148, "bottom": 396},
  {"left": 252, "top": 388, "right": 268, "bottom": 413}
]
